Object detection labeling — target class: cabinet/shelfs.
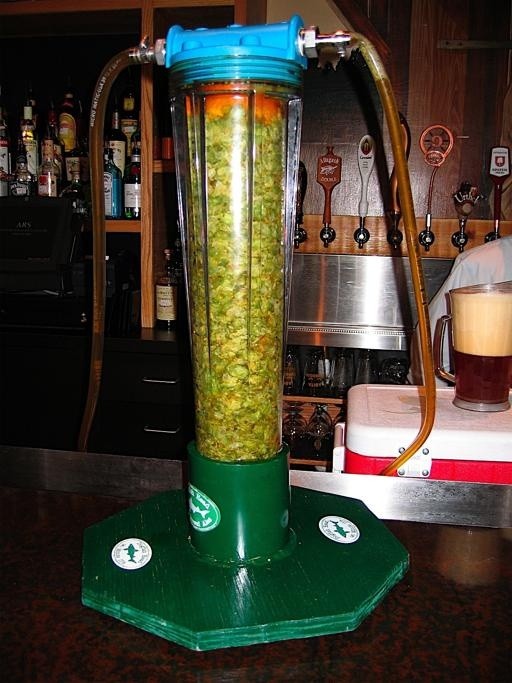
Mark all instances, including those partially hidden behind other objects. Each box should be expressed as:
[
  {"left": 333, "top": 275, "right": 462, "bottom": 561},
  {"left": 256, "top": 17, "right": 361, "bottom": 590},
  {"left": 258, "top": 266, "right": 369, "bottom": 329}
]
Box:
[{"left": 1, "top": 0, "right": 268, "bottom": 460}]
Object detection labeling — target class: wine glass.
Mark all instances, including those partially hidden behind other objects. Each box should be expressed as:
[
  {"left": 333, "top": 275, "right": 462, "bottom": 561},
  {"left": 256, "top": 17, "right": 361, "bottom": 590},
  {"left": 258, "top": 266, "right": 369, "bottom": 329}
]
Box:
[{"left": 282, "top": 401, "right": 347, "bottom": 460}]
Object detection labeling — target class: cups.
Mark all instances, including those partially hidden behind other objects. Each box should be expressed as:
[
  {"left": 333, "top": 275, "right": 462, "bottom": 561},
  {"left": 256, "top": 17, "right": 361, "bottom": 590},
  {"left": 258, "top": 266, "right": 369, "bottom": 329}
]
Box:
[{"left": 435, "top": 279, "right": 511, "bottom": 413}]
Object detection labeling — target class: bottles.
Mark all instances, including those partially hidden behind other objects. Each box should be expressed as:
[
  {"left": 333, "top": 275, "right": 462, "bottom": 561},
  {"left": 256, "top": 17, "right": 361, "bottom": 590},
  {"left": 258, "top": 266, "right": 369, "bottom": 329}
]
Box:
[
  {"left": 154, "top": 235, "right": 184, "bottom": 332},
  {"left": 284, "top": 345, "right": 381, "bottom": 397},
  {"left": 1, "top": 65, "right": 141, "bottom": 220}
]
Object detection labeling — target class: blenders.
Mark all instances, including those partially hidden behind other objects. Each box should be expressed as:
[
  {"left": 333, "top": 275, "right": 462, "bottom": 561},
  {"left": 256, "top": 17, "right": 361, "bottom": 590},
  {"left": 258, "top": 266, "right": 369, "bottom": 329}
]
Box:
[{"left": 78, "top": 12, "right": 411, "bottom": 656}]
